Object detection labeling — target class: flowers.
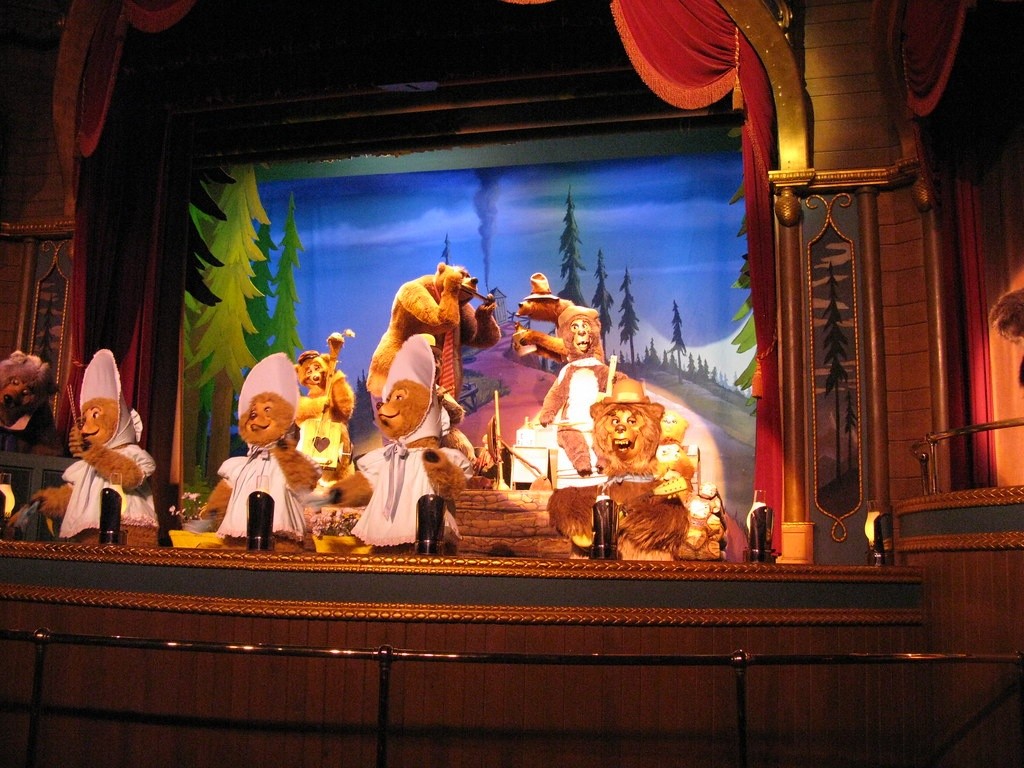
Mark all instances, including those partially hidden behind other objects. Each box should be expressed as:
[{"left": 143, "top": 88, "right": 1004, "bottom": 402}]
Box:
[
  {"left": 309, "top": 507, "right": 359, "bottom": 540},
  {"left": 169, "top": 491, "right": 207, "bottom": 530}
]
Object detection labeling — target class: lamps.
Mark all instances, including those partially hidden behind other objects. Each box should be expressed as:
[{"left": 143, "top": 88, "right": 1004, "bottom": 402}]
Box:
[
  {"left": 413, "top": 494, "right": 447, "bottom": 555},
  {"left": 588, "top": 500, "right": 619, "bottom": 561},
  {"left": 98, "top": 488, "right": 123, "bottom": 546},
  {"left": 246, "top": 491, "right": 275, "bottom": 551},
  {"left": 743, "top": 507, "right": 775, "bottom": 562}
]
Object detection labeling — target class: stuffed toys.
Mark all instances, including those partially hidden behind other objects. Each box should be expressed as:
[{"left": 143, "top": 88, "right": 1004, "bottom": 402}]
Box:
[
  {"left": 291, "top": 329, "right": 356, "bottom": 480},
  {"left": 200, "top": 353, "right": 323, "bottom": 547},
  {"left": 550, "top": 377, "right": 694, "bottom": 560},
  {"left": 516, "top": 272, "right": 575, "bottom": 362},
  {"left": 989, "top": 287, "right": 1024, "bottom": 386},
  {"left": 540, "top": 306, "right": 632, "bottom": 475},
  {"left": 0, "top": 350, "right": 64, "bottom": 456},
  {"left": 367, "top": 262, "right": 500, "bottom": 461},
  {"left": 330, "top": 334, "right": 475, "bottom": 553},
  {"left": 32, "top": 349, "right": 161, "bottom": 547}
]
